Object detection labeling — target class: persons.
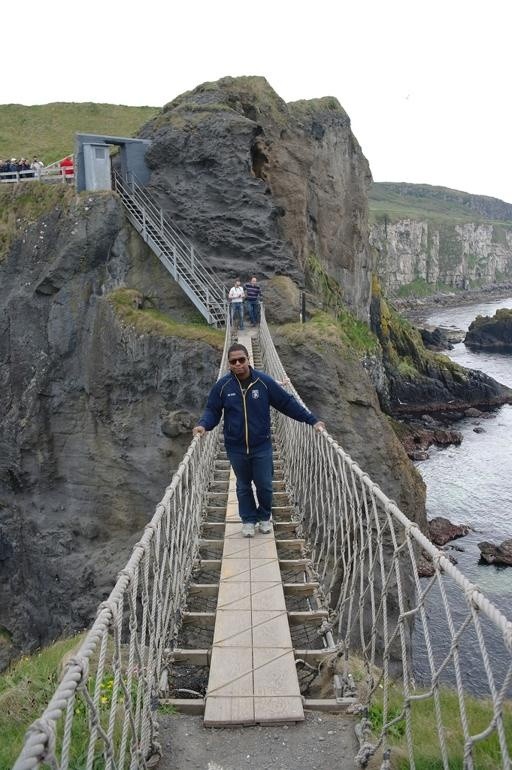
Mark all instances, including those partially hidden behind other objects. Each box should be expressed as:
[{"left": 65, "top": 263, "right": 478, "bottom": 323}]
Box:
[
  {"left": 193, "top": 344, "right": 325, "bottom": 537},
  {"left": 243, "top": 276, "right": 263, "bottom": 327},
  {"left": 0, "top": 155, "right": 74, "bottom": 184},
  {"left": 228, "top": 279, "right": 246, "bottom": 330}
]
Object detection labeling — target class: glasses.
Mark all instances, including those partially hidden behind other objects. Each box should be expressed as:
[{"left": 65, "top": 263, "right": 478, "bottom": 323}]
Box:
[{"left": 229, "top": 356, "right": 248, "bottom": 365}]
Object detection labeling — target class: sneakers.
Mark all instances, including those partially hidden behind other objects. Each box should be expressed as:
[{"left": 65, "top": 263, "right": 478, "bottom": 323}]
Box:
[
  {"left": 241, "top": 522, "right": 255, "bottom": 537},
  {"left": 258, "top": 519, "right": 272, "bottom": 533}
]
